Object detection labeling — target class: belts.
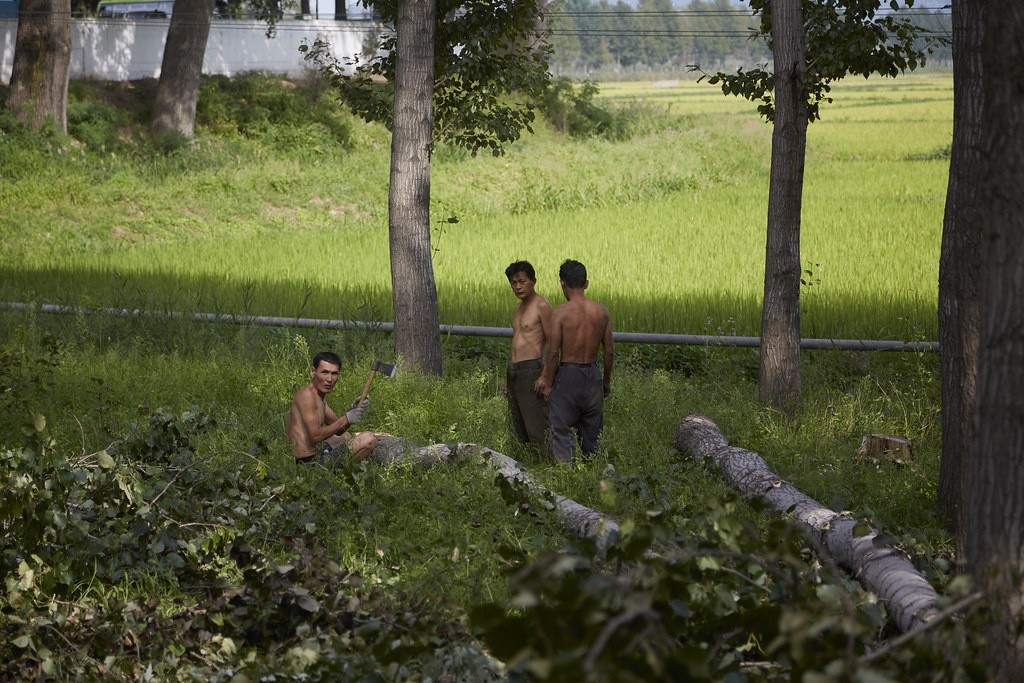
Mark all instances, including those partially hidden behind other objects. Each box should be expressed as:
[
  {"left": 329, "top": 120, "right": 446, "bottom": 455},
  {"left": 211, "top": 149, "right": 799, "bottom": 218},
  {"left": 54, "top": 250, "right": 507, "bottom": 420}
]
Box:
[
  {"left": 508, "top": 359, "right": 544, "bottom": 370},
  {"left": 559, "top": 362, "right": 598, "bottom": 368},
  {"left": 296, "top": 453, "right": 318, "bottom": 465}
]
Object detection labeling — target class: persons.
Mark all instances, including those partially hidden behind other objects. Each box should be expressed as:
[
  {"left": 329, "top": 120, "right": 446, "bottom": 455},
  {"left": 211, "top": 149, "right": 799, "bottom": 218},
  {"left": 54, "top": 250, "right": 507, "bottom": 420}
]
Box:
[
  {"left": 287, "top": 352, "right": 379, "bottom": 468},
  {"left": 502, "top": 261, "right": 553, "bottom": 443},
  {"left": 542, "top": 259, "right": 615, "bottom": 465}
]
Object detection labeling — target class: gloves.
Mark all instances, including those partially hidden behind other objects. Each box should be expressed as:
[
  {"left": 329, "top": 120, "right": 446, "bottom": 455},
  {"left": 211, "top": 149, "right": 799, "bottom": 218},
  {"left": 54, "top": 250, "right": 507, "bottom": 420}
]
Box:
[
  {"left": 351, "top": 394, "right": 370, "bottom": 410},
  {"left": 346, "top": 408, "right": 364, "bottom": 424}
]
